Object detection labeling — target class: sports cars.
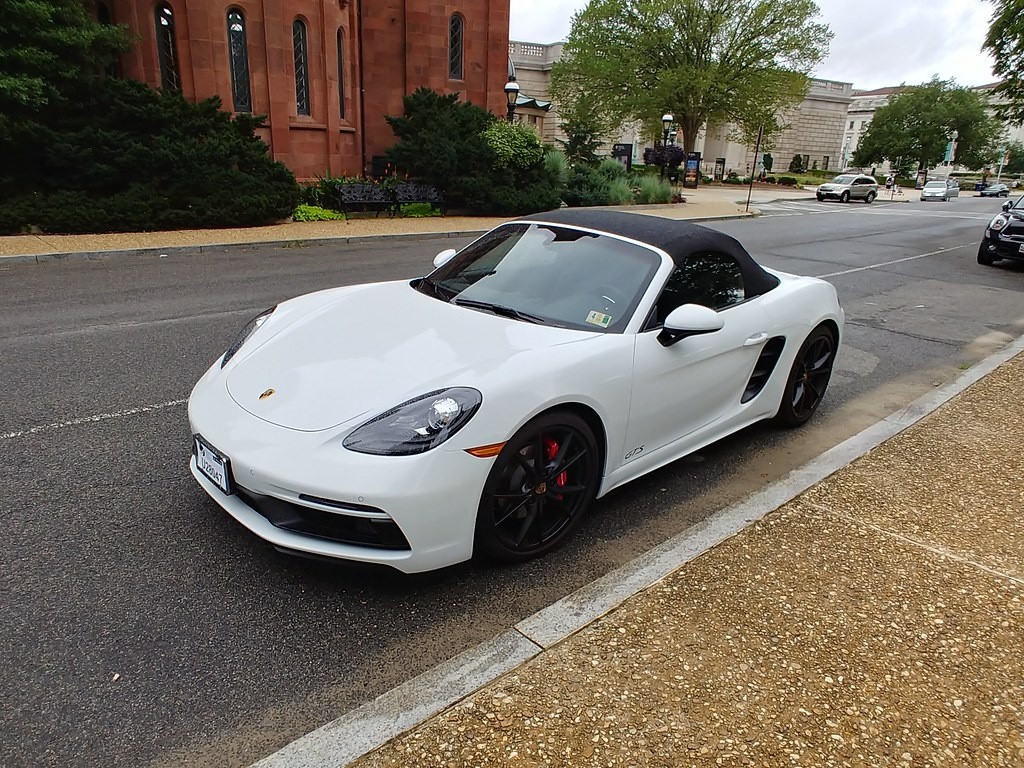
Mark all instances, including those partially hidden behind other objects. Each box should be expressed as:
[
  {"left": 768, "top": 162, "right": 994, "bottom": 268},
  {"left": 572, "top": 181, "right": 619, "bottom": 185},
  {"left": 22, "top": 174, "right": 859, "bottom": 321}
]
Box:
[{"left": 188, "top": 207, "right": 846, "bottom": 575}]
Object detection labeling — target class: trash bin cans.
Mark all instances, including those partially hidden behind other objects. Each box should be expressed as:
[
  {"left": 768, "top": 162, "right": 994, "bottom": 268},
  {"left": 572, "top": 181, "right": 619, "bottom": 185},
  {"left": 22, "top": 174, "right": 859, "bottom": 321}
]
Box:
[
  {"left": 975, "top": 184, "right": 982, "bottom": 191},
  {"left": 372, "top": 155, "right": 394, "bottom": 179},
  {"left": 982, "top": 184, "right": 987, "bottom": 191}
]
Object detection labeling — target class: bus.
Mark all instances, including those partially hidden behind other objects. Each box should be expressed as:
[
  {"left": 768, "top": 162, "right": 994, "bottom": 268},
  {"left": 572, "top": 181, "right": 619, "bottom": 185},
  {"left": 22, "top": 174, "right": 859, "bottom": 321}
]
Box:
[{"left": 947, "top": 172, "right": 1024, "bottom": 189}]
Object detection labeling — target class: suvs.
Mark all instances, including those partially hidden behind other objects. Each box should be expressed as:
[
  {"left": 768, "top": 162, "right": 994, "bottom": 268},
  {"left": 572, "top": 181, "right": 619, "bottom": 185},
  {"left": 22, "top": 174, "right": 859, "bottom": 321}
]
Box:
[{"left": 815, "top": 173, "right": 878, "bottom": 203}]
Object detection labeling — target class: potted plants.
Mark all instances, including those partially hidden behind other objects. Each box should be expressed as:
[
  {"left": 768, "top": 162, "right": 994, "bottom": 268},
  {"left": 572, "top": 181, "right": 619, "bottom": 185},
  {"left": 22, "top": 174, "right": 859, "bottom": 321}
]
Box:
[{"left": 696, "top": 170, "right": 798, "bottom": 187}]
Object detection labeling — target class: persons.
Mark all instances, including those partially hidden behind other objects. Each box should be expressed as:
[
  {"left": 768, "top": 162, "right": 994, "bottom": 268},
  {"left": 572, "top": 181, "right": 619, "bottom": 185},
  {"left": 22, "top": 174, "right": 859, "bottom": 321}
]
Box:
[
  {"left": 884, "top": 174, "right": 902, "bottom": 193},
  {"left": 947, "top": 177, "right": 958, "bottom": 201},
  {"left": 759, "top": 166, "right": 766, "bottom": 179},
  {"left": 745, "top": 163, "right": 751, "bottom": 176},
  {"left": 1011, "top": 180, "right": 1017, "bottom": 192}
]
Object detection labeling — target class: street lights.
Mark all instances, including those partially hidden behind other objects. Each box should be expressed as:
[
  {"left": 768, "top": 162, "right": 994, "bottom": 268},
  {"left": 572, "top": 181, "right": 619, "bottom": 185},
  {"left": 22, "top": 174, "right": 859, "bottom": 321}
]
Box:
[
  {"left": 944, "top": 131, "right": 958, "bottom": 178},
  {"left": 504, "top": 76, "right": 519, "bottom": 123},
  {"left": 841, "top": 137, "right": 850, "bottom": 172},
  {"left": 670, "top": 127, "right": 678, "bottom": 144},
  {"left": 995, "top": 136, "right": 1010, "bottom": 184},
  {"left": 661, "top": 112, "right": 673, "bottom": 184}
]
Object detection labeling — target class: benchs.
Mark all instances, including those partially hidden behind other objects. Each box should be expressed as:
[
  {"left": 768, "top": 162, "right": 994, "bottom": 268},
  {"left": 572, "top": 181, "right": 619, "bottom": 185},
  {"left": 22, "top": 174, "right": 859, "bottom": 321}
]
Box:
[{"left": 335, "top": 181, "right": 454, "bottom": 225}]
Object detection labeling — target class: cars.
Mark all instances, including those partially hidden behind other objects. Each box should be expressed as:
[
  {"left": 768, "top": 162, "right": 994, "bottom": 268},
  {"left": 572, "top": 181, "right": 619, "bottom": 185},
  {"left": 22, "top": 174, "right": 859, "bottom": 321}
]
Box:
[
  {"left": 978, "top": 193, "right": 1023, "bottom": 267},
  {"left": 980, "top": 185, "right": 1010, "bottom": 196},
  {"left": 920, "top": 181, "right": 960, "bottom": 201}
]
